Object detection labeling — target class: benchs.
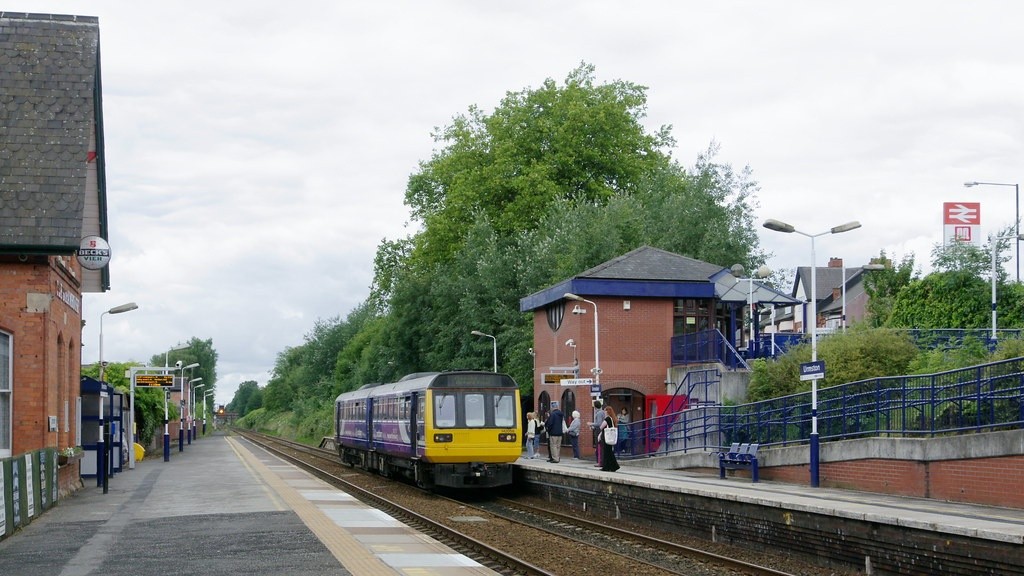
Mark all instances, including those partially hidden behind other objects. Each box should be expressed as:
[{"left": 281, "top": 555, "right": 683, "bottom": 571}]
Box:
[{"left": 709, "top": 443, "right": 759, "bottom": 483}]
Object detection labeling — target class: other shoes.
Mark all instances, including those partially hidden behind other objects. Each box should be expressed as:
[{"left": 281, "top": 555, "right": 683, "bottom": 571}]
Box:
[
  {"left": 622, "top": 450, "right": 625, "bottom": 453},
  {"left": 599, "top": 468, "right": 610, "bottom": 471},
  {"left": 551, "top": 460, "right": 558, "bottom": 463},
  {"left": 571, "top": 458, "right": 577, "bottom": 460},
  {"left": 594, "top": 464, "right": 602, "bottom": 467},
  {"left": 611, "top": 467, "right": 619, "bottom": 472},
  {"left": 547, "top": 459, "right": 552, "bottom": 462}
]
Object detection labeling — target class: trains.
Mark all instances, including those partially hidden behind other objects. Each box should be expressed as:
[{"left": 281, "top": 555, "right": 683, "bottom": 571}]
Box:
[{"left": 333, "top": 371, "right": 522, "bottom": 489}]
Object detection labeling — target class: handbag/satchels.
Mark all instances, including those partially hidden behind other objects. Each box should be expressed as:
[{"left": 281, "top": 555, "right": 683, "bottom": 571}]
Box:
[{"left": 604, "top": 417, "right": 618, "bottom": 445}]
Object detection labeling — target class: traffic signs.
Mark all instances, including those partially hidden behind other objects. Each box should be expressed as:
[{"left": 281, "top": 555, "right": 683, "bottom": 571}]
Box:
[{"left": 560, "top": 378, "right": 603, "bottom": 407}]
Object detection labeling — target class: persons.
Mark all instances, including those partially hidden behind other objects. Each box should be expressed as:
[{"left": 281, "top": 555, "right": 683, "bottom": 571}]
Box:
[
  {"left": 545, "top": 410, "right": 553, "bottom": 462},
  {"left": 599, "top": 406, "right": 620, "bottom": 472},
  {"left": 532, "top": 412, "right": 544, "bottom": 458},
  {"left": 587, "top": 401, "right": 605, "bottom": 467},
  {"left": 544, "top": 401, "right": 564, "bottom": 463},
  {"left": 566, "top": 411, "right": 580, "bottom": 459},
  {"left": 617, "top": 407, "right": 630, "bottom": 453},
  {"left": 525, "top": 412, "right": 535, "bottom": 459}
]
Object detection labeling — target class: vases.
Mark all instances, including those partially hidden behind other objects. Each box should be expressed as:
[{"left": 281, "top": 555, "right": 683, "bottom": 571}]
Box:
[{"left": 59, "top": 452, "right": 84, "bottom": 466}]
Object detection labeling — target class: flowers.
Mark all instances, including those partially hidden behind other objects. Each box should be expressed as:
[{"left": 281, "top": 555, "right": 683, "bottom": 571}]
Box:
[{"left": 59, "top": 446, "right": 82, "bottom": 456}]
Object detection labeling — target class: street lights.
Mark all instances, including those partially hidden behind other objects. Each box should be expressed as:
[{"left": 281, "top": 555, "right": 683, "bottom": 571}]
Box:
[
  {"left": 204, "top": 393, "right": 212, "bottom": 431},
  {"left": 193, "top": 384, "right": 205, "bottom": 440},
  {"left": 965, "top": 181, "right": 1019, "bottom": 283},
  {"left": 988, "top": 234, "right": 1024, "bottom": 354},
  {"left": 470, "top": 331, "right": 497, "bottom": 373},
  {"left": 164, "top": 344, "right": 190, "bottom": 461},
  {"left": 188, "top": 377, "right": 202, "bottom": 444},
  {"left": 96, "top": 302, "right": 138, "bottom": 486},
  {"left": 763, "top": 218, "right": 862, "bottom": 487},
  {"left": 203, "top": 388, "right": 213, "bottom": 434},
  {"left": 179, "top": 362, "right": 200, "bottom": 452},
  {"left": 563, "top": 293, "right": 603, "bottom": 417},
  {"left": 731, "top": 263, "right": 770, "bottom": 358}
]
